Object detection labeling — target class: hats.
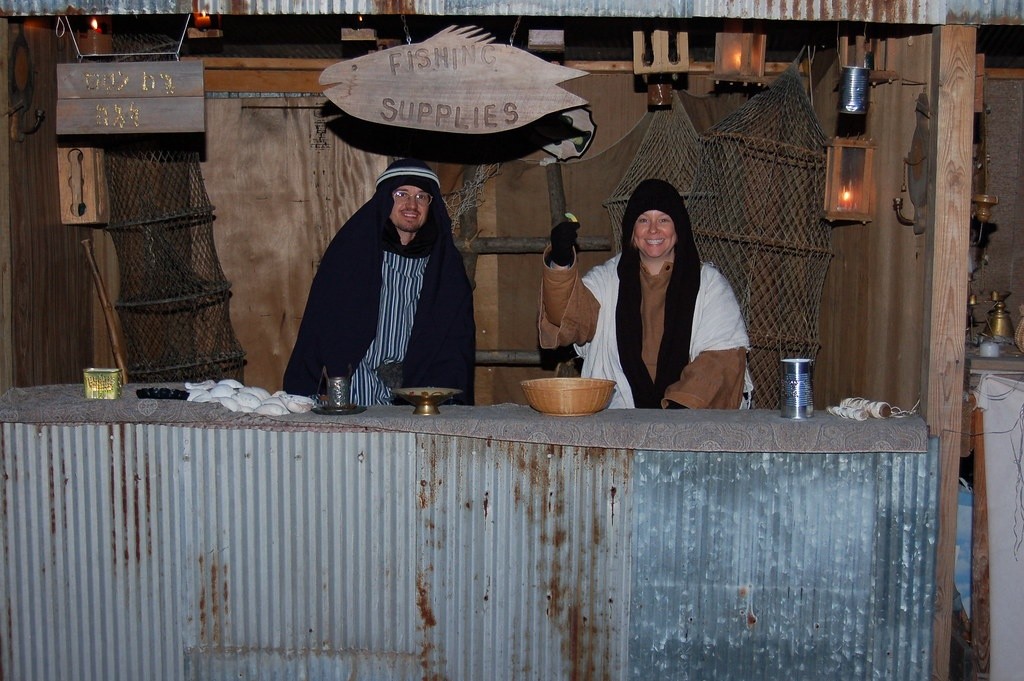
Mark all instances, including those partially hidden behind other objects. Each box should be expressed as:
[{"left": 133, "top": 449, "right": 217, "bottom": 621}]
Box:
[{"left": 375, "top": 158, "right": 441, "bottom": 197}]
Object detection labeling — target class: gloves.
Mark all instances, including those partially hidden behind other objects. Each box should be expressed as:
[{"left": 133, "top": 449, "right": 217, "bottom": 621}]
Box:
[
  {"left": 665, "top": 400, "right": 687, "bottom": 409},
  {"left": 549, "top": 221, "right": 581, "bottom": 266}
]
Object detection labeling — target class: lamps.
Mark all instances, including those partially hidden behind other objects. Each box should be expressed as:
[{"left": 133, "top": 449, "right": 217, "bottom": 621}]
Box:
[
  {"left": 715, "top": 32, "right": 766, "bottom": 85},
  {"left": 817, "top": 136, "right": 877, "bottom": 225}
]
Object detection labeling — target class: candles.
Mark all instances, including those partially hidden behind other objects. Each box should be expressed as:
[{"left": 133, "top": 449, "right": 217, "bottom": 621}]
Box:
[
  {"left": 197, "top": 10, "right": 211, "bottom": 29},
  {"left": 980, "top": 340, "right": 1000, "bottom": 357}
]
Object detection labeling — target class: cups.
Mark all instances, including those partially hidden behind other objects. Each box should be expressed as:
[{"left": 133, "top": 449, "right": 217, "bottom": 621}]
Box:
[{"left": 779, "top": 357, "right": 814, "bottom": 420}]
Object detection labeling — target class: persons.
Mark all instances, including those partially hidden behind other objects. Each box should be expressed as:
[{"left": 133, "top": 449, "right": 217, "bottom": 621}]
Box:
[
  {"left": 536, "top": 178, "right": 753, "bottom": 412},
  {"left": 282, "top": 160, "right": 476, "bottom": 406}
]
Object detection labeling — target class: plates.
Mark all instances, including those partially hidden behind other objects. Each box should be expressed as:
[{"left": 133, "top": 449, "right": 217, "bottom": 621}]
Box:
[{"left": 311, "top": 405, "right": 367, "bottom": 415}]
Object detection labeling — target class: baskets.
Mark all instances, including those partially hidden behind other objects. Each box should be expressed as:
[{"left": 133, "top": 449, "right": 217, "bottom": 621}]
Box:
[{"left": 519, "top": 377, "right": 617, "bottom": 417}]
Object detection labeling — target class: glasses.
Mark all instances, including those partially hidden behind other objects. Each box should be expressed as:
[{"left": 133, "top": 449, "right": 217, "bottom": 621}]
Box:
[{"left": 392, "top": 190, "right": 433, "bottom": 207}]
